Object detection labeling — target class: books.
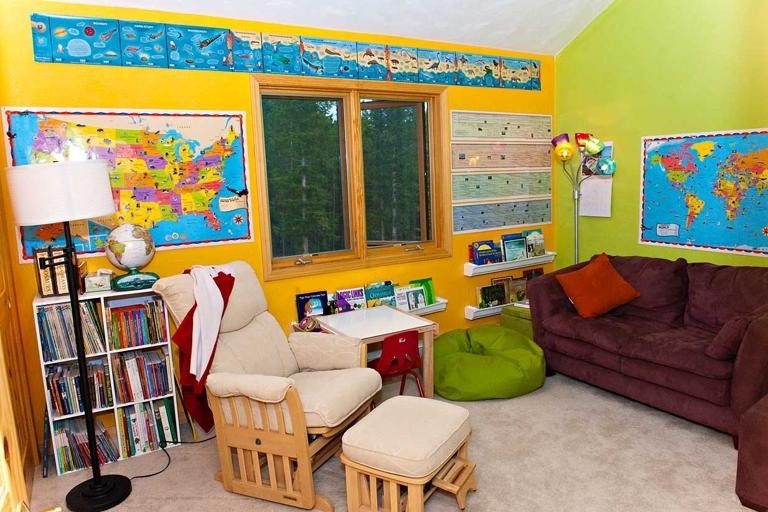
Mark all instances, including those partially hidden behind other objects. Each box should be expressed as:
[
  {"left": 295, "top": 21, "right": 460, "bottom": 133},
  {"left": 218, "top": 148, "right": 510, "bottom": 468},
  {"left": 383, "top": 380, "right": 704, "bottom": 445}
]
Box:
[
  {"left": 468, "top": 228, "right": 545, "bottom": 309},
  {"left": 296, "top": 278, "right": 436, "bottom": 333},
  {"left": 32, "top": 246, "right": 177, "bottom": 476}
]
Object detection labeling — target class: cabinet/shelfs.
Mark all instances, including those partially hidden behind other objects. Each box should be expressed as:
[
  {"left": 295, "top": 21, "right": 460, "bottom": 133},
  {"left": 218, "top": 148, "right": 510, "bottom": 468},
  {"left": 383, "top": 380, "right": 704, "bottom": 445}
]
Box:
[
  {"left": 463, "top": 230, "right": 557, "bottom": 322},
  {"left": 33, "top": 289, "right": 181, "bottom": 479}
]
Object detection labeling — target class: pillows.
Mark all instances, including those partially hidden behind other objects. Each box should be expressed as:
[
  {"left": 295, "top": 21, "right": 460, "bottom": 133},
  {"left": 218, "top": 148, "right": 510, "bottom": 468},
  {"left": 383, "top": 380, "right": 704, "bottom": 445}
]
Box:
[
  {"left": 555, "top": 252, "right": 640, "bottom": 320},
  {"left": 705, "top": 303, "right": 767, "bottom": 361}
]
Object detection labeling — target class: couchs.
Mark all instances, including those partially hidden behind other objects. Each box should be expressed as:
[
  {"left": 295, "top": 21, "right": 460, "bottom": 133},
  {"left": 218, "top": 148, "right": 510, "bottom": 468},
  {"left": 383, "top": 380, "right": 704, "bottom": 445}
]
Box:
[{"left": 526, "top": 254, "right": 768, "bottom": 450}]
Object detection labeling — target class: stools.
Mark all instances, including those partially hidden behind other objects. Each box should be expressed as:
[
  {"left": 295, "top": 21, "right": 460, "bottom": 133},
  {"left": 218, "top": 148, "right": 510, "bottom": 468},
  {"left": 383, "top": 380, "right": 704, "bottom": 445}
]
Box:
[
  {"left": 736, "top": 395, "right": 768, "bottom": 511},
  {"left": 339, "top": 395, "right": 478, "bottom": 512}
]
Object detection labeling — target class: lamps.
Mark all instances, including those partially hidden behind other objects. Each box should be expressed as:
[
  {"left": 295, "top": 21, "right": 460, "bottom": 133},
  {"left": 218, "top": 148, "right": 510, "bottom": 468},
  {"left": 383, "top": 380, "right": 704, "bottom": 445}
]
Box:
[
  {"left": 8, "top": 161, "right": 132, "bottom": 512},
  {"left": 551, "top": 131, "right": 616, "bottom": 264}
]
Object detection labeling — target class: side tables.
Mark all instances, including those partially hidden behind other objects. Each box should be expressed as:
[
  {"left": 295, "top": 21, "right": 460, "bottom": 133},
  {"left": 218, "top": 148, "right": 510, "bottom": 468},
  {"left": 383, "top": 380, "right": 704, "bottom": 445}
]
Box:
[{"left": 316, "top": 305, "right": 440, "bottom": 395}]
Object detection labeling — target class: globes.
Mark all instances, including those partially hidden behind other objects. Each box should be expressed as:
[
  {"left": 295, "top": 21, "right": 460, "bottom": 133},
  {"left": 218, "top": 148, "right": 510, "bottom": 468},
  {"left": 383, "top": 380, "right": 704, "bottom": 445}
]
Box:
[{"left": 104, "top": 222, "right": 159, "bottom": 291}]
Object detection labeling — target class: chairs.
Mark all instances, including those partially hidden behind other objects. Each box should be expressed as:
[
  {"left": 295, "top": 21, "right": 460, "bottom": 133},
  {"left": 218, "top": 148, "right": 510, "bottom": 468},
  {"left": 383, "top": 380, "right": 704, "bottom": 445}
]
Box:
[
  {"left": 153, "top": 261, "right": 382, "bottom": 511},
  {"left": 367, "top": 330, "right": 431, "bottom": 397}
]
